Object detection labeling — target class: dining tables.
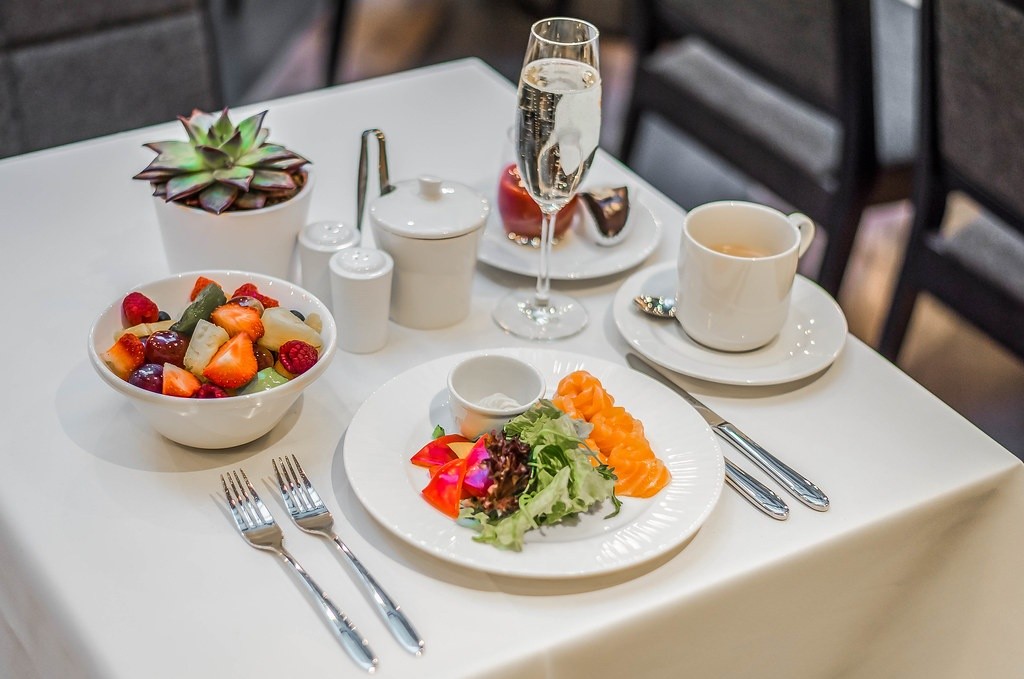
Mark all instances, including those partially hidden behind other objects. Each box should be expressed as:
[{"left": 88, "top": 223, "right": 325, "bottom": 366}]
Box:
[{"left": 0, "top": 54, "right": 1024, "bottom": 679}]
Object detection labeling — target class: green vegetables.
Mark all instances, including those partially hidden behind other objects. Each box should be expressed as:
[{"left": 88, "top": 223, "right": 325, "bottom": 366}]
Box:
[{"left": 459, "top": 397, "right": 622, "bottom": 551}]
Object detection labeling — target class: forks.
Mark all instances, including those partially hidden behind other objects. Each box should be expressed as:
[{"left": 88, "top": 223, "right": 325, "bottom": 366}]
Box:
[
  {"left": 220, "top": 469, "right": 379, "bottom": 673},
  {"left": 273, "top": 454, "right": 425, "bottom": 657}
]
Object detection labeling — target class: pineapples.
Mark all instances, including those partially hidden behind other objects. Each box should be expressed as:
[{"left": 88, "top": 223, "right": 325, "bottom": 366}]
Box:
[{"left": 181, "top": 318, "right": 229, "bottom": 382}]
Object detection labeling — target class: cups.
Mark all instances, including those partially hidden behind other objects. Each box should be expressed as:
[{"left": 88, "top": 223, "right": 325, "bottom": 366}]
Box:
[
  {"left": 497, "top": 127, "right": 579, "bottom": 247},
  {"left": 678, "top": 202, "right": 814, "bottom": 350},
  {"left": 299, "top": 221, "right": 361, "bottom": 315},
  {"left": 328, "top": 248, "right": 393, "bottom": 353},
  {"left": 373, "top": 176, "right": 488, "bottom": 328}
]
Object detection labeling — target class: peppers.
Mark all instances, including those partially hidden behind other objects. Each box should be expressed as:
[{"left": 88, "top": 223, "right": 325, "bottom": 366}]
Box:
[{"left": 410, "top": 433, "right": 488, "bottom": 518}]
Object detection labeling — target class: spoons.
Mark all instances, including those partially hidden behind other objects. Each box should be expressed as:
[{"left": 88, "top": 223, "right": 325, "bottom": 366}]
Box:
[{"left": 634, "top": 295, "right": 678, "bottom": 318}]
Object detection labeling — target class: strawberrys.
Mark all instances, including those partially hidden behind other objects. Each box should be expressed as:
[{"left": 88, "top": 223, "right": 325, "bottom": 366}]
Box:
[{"left": 103, "top": 276, "right": 279, "bottom": 397}]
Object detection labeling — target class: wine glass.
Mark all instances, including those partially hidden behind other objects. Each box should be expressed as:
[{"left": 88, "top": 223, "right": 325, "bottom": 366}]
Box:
[{"left": 493, "top": 18, "right": 602, "bottom": 339}]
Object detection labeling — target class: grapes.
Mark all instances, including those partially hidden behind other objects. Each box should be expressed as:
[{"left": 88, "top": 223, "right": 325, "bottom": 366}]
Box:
[{"left": 128, "top": 329, "right": 275, "bottom": 392}]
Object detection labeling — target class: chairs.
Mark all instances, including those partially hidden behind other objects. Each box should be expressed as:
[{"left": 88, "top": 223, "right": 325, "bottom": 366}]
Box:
[{"left": 0, "top": 0, "right": 1024, "bottom": 371}]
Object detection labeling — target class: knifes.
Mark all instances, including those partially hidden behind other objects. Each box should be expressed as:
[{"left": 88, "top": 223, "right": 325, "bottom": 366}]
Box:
[{"left": 626, "top": 353, "right": 830, "bottom": 520}]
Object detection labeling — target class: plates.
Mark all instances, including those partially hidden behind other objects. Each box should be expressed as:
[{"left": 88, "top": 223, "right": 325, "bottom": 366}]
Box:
[
  {"left": 478, "top": 175, "right": 659, "bottom": 280},
  {"left": 614, "top": 261, "right": 847, "bottom": 386},
  {"left": 343, "top": 348, "right": 725, "bottom": 579}
]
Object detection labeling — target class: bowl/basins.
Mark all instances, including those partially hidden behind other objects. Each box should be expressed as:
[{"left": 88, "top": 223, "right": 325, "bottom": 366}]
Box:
[
  {"left": 447, "top": 355, "right": 546, "bottom": 441},
  {"left": 87, "top": 270, "right": 338, "bottom": 448}
]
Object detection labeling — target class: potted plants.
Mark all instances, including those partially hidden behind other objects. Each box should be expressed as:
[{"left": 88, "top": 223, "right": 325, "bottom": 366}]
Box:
[{"left": 132, "top": 105, "right": 316, "bottom": 282}]
